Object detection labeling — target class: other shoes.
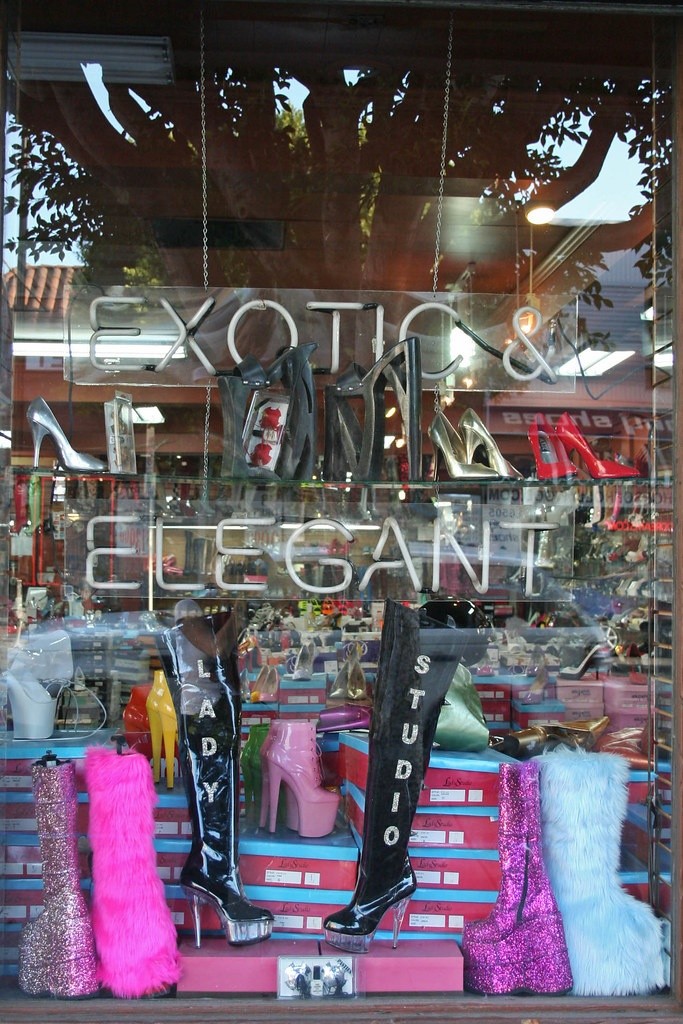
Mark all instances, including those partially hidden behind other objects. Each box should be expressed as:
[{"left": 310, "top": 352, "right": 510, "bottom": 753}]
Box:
[{"left": 236, "top": 603, "right": 648, "bottom": 708}]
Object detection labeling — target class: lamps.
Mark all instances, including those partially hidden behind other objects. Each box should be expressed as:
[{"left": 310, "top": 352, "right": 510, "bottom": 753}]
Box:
[
  {"left": 524, "top": 201, "right": 555, "bottom": 224},
  {"left": 6, "top": 30, "right": 176, "bottom": 86}
]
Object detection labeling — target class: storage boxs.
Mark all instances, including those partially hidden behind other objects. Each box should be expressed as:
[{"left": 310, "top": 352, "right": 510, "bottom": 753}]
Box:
[{"left": 0, "top": 643, "right": 675, "bottom": 995}]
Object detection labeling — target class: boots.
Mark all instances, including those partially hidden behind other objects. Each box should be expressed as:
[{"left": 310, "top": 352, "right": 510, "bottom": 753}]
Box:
[
  {"left": 155, "top": 611, "right": 275, "bottom": 948},
  {"left": 87, "top": 748, "right": 181, "bottom": 998},
  {"left": 538, "top": 754, "right": 661, "bottom": 997},
  {"left": 324, "top": 598, "right": 469, "bottom": 952},
  {"left": 21, "top": 759, "right": 99, "bottom": 996},
  {"left": 463, "top": 762, "right": 572, "bottom": 994}
]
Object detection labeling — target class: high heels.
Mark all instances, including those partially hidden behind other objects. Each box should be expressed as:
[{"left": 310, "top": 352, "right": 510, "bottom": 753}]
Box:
[
  {"left": 428, "top": 411, "right": 503, "bottom": 481},
  {"left": 27, "top": 397, "right": 106, "bottom": 471},
  {"left": 556, "top": 412, "right": 642, "bottom": 477},
  {"left": 241, "top": 722, "right": 288, "bottom": 827},
  {"left": 459, "top": 406, "right": 524, "bottom": 480},
  {"left": 147, "top": 671, "right": 180, "bottom": 789},
  {"left": 123, "top": 686, "right": 180, "bottom": 761},
  {"left": 265, "top": 721, "right": 341, "bottom": 838},
  {"left": 258, "top": 722, "right": 300, "bottom": 833},
  {"left": 527, "top": 412, "right": 579, "bottom": 480}
]
